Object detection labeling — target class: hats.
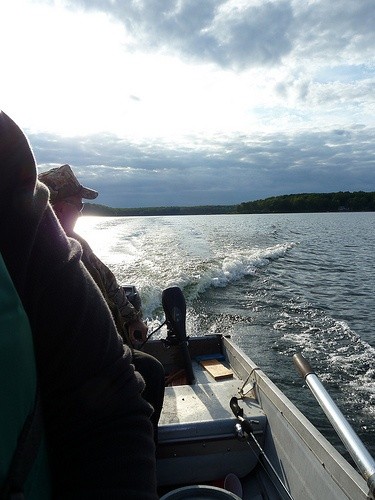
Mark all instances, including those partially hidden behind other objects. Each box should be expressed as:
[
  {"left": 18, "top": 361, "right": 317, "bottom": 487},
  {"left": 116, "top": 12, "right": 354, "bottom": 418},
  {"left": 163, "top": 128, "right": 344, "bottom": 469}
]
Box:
[{"left": 38, "top": 163, "right": 99, "bottom": 204}]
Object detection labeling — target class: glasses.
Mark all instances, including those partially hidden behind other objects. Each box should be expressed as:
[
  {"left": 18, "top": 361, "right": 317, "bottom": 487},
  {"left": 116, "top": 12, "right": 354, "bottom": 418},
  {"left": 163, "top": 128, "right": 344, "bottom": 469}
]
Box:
[{"left": 60, "top": 199, "right": 85, "bottom": 212}]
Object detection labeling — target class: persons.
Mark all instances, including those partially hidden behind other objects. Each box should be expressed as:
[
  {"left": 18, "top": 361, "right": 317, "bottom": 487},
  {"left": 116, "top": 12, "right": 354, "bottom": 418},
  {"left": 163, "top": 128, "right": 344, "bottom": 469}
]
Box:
[
  {"left": 0, "top": 108, "right": 159, "bottom": 500},
  {"left": 35, "top": 161, "right": 150, "bottom": 359}
]
